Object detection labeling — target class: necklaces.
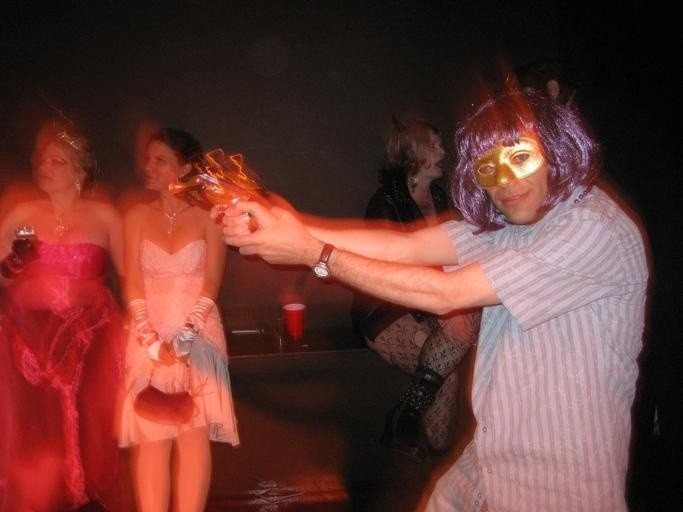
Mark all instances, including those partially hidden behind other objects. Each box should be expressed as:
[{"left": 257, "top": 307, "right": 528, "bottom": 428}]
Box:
[
  {"left": 52, "top": 202, "right": 69, "bottom": 235},
  {"left": 573, "top": 182, "right": 595, "bottom": 205},
  {"left": 156, "top": 198, "right": 191, "bottom": 237}
]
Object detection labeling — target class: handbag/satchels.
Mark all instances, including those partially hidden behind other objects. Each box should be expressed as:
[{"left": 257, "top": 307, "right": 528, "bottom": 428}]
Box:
[{"left": 134, "top": 386, "right": 194, "bottom": 427}]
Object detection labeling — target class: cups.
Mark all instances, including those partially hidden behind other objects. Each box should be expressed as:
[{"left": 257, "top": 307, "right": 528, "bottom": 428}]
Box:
[
  {"left": 281, "top": 303, "right": 305, "bottom": 339},
  {"left": 11, "top": 225, "right": 39, "bottom": 262}
]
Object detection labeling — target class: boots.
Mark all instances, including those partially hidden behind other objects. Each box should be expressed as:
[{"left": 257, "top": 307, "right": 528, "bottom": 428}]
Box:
[{"left": 384, "top": 367, "right": 443, "bottom": 460}]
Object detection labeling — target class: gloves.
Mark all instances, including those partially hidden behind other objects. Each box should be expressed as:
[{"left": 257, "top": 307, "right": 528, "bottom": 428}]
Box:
[{"left": 0, "top": 239, "right": 39, "bottom": 278}]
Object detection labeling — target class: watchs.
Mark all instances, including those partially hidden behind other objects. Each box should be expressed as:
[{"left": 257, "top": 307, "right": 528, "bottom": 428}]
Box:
[{"left": 310, "top": 243, "right": 335, "bottom": 288}]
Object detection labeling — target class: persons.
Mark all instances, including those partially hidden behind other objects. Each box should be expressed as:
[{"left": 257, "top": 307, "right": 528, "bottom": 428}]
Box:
[
  {"left": 0, "top": 123, "right": 131, "bottom": 512},
  {"left": 209, "top": 88, "right": 650, "bottom": 512},
  {"left": 117, "top": 128, "right": 242, "bottom": 512},
  {"left": 349, "top": 118, "right": 484, "bottom": 465}
]
